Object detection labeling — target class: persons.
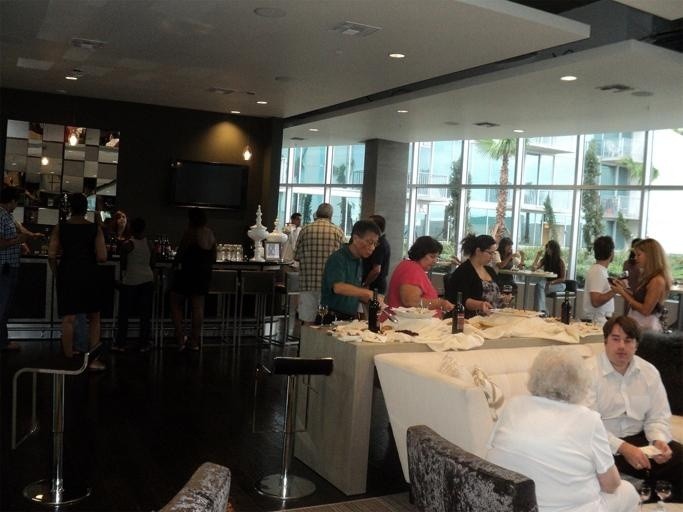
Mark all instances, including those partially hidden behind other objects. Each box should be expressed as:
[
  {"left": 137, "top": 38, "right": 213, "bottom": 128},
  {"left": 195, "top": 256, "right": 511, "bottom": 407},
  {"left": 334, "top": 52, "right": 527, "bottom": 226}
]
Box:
[
  {"left": 171, "top": 207, "right": 217, "bottom": 351},
  {"left": 48, "top": 192, "right": 107, "bottom": 371},
  {"left": 581, "top": 235, "right": 626, "bottom": 325},
  {"left": 285, "top": 204, "right": 565, "bottom": 324},
  {"left": 112, "top": 216, "right": 157, "bottom": 352},
  {"left": 106, "top": 209, "right": 130, "bottom": 242},
  {"left": 623, "top": 238, "right": 642, "bottom": 316},
  {"left": 610, "top": 238, "right": 672, "bottom": 331},
  {"left": 488, "top": 347, "right": 641, "bottom": 511},
  {"left": 0, "top": 186, "right": 45, "bottom": 349},
  {"left": 582, "top": 316, "right": 683, "bottom": 503}
]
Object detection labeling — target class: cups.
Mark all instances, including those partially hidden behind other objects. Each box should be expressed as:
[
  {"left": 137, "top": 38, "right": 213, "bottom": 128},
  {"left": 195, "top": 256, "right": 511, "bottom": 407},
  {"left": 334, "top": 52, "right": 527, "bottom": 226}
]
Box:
[{"left": 421, "top": 297, "right": 432, "bottom": 314}]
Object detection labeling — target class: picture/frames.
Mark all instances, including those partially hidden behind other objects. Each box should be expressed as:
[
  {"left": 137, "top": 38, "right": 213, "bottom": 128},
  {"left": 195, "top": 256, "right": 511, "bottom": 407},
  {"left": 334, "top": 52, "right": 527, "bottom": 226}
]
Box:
[{"left": 264, "top": 241, "right": 281, "bottom": 262}]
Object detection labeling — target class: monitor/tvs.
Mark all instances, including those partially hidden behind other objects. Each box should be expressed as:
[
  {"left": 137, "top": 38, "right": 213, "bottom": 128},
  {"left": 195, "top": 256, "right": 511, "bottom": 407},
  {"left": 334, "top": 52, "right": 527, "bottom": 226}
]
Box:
[{"left": 168, "top": 159, "right": 248, "bottom": 213}]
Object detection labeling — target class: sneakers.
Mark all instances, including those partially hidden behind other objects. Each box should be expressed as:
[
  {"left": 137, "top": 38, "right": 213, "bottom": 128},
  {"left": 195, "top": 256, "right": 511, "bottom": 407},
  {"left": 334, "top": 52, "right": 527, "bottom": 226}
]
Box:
[{"left": 111, "top": 342, "right": 150, "bottom": 352}]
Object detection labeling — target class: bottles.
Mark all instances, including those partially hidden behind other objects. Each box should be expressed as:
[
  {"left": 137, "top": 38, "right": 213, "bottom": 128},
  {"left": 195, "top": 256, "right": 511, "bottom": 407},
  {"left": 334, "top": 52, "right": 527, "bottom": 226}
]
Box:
[
  {"left": 149, "top": 234, "right": 172, "bottom": 257},
  {"left": 368, "top": 287, "right": 381, "bottom": 333},
  {"left": 40, "top": 227, "right": 50, "bottom": 255},
  {"left": 216, "top": 243, "right": 244, "bottom": 262},
  {"left": 451, "top": 292, "right": 465, "bottom": 334},
  {"left": 561, "top": 291, "right": 571, "bottom": 325},
  {"left": 111, "top": 236, "right": 117, "bottom": 255}
]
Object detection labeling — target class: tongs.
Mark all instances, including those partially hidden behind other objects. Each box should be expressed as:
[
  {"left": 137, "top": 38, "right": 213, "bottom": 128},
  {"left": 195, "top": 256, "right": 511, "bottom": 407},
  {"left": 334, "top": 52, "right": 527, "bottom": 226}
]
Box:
[{"left": 380, "top": 305, "right": 399, "bottom": 323}]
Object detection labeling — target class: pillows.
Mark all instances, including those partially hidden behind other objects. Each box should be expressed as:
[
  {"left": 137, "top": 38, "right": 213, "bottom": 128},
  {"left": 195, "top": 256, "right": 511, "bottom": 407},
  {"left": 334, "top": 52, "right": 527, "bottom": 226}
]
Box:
[
  {"left": 473, "top": 368, "right": 504, "bottom": 422},
  {"left": 438, "top": 353, "right": 473, "bottom": 383}
]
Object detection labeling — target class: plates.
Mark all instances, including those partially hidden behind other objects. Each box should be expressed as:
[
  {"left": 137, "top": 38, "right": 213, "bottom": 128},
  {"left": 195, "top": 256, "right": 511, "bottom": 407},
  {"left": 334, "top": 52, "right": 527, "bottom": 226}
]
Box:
[
  {"left": 390, "top": 306, "right": 439, "bottom": 319},
  {"left": 490, "top": 307, "right": 546, "bottom": 318}
]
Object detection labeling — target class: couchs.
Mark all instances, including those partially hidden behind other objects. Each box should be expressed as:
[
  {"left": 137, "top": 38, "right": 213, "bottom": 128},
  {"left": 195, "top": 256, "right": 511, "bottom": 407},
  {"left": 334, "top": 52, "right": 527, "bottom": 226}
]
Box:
[{"left": 373, "top": 342, "right": 683, "bottom": 504}]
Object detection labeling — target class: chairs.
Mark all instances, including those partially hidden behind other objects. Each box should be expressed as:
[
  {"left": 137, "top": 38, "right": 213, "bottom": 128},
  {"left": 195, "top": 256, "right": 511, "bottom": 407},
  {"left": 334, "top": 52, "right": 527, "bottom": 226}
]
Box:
[
  {"left": 160, "top": 462, "right": 232, "bottom": 511},
  {"left": 406, "top": 425, "right": 535, "bottom": 511}
]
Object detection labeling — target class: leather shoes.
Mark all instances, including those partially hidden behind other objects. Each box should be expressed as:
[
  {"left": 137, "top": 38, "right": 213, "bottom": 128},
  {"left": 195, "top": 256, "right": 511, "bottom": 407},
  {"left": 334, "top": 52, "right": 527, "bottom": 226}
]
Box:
[
  {"left": 179, "top": 335, "right": 199, "bottom": 351},
  {"left": 0, "top": 342, "right": 20, "bottom": 350}
]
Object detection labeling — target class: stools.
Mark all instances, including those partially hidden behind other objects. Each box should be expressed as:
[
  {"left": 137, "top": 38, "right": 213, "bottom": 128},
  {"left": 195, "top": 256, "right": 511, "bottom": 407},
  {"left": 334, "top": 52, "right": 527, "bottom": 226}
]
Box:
[
  {"left": 252, "top": 356, "right": 334, "bottom": 501},
  {"left": 545, "top": 280, "right": 576, "bottom": 318},
  {"left": 10, "top": 342, "right": 103, "bottom": 507},
  {"left": 151, "top": 270, "right": 300, "bottom": 350}
]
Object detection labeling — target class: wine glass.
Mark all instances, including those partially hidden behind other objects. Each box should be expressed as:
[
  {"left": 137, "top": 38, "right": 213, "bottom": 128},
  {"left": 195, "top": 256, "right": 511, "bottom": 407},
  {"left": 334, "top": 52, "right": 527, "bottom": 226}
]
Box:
[
  {"left": 318, "top": 305, "right": 329, "bottom": 329},
  {"left": 502, "top": 284, "right": 514, "bottom": 304},
  {"left": 636, "top": 484, "right": 652, "bottom": 512},
  {"left": 521, "top": 262, "right": 526, "bottom": 273},
  {"left": 619, "top": 270, "right": 632, "bottom": 290},
  {"left": 654, "top": 480, "right": 672, "bottom": 511},
  {"left": 534, "top": 263, "right": 539, "bottom": 274}
]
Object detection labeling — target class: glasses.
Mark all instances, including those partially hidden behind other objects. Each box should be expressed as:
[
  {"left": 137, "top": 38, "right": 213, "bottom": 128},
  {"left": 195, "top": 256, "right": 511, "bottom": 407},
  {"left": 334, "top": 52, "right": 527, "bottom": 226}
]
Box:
[
  {"left": 361, "top": 239, "right": 381, "bottom": 247},
  {"left": 480, "top": 249, "right": 497, "bottom": 256}
]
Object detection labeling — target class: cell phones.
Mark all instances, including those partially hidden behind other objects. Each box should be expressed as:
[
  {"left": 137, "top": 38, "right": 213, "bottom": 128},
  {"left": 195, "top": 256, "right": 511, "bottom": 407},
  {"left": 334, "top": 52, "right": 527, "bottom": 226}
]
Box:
[{"left": 607, "top": 278, "right": 614, "bottom": 286}]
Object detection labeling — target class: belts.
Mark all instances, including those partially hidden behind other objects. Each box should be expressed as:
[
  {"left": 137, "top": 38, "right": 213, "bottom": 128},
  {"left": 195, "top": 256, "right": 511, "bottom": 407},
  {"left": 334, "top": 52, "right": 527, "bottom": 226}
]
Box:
[{"left": 328, "top": 310, "right": 356, "bottom": 320}]
